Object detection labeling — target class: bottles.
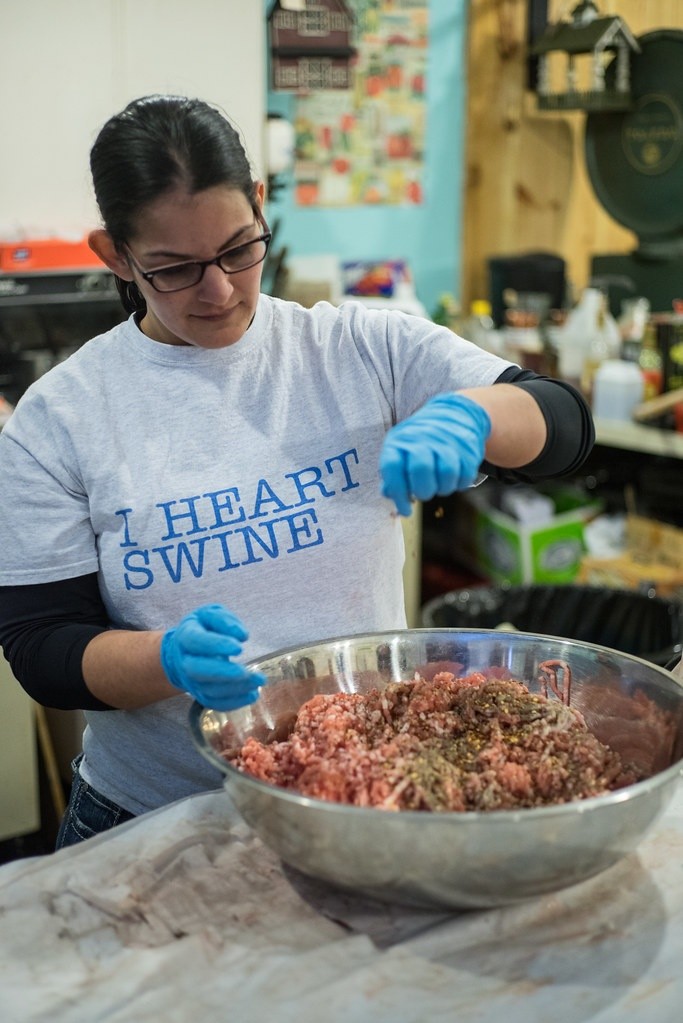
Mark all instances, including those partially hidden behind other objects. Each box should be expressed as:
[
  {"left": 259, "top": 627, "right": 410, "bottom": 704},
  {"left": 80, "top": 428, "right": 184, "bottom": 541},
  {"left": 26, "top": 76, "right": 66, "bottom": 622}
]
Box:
[{"left": 441, "top": 291, "right": 664, "bottom": 431}]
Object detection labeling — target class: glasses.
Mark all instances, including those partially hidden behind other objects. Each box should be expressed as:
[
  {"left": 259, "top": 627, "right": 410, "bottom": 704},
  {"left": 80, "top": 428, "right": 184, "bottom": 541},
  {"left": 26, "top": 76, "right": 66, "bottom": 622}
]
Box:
[{"left": 124, "top": 196, "right": 274, "bottom": 293}]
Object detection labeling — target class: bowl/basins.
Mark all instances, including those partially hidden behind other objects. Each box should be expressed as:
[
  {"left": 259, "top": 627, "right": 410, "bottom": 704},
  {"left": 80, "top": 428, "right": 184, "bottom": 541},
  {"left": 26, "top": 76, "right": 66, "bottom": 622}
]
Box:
[{"left": 187, "top": 628, "right": 683, "bottom": 915}]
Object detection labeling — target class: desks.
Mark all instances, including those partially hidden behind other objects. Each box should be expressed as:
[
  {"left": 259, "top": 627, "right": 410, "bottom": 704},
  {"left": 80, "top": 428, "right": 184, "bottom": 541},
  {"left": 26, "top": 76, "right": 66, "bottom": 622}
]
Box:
[{"left": 0, "top": 661, "right": 682, "bottom": 1023}]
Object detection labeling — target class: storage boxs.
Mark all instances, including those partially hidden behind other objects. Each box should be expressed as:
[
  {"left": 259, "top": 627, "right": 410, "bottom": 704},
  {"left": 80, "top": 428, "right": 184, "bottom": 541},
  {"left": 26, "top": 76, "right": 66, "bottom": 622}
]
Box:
[{"left": 468, "top": 486, "right": 604, "bottom": 588}]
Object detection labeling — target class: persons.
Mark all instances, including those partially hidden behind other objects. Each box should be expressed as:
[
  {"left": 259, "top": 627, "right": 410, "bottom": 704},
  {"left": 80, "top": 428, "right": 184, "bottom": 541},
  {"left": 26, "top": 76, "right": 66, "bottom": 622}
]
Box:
[{"left": 0, "top": 94, "right": 596, "bottom": 853}]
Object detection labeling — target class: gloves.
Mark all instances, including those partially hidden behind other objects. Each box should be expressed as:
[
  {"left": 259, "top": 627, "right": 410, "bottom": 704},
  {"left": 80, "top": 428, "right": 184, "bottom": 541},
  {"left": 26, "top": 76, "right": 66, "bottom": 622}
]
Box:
[
  {"left": 161, "top": 601, "right": 267, "bottom": 713},
  {"left": 380, "top": 390, "right": 493, "bottom": 518}
]
